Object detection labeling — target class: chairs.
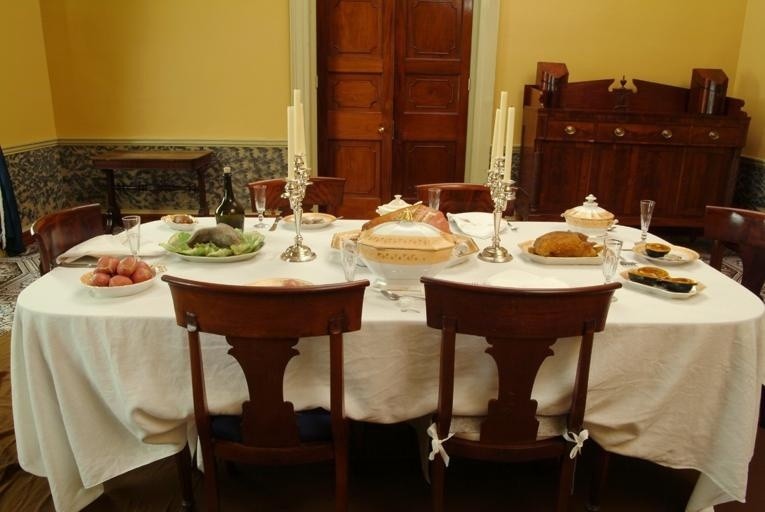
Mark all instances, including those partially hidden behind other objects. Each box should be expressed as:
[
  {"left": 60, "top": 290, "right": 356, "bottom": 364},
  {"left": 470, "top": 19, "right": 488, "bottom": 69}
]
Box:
[
  {"left": 159, "top": 269, "right": 371, "bottom": 512},
  {"left": 698, "top": 204, "right": 764, "bottom": 302},
  {"left": 414, "top": 182, "right": 521, "bottom": 221},
  {"left": 30, "top": 203, "right": 107, "bottom": 277},
  {"left": 248, "top": 175, "right": 347, "bottom": 214},
  {"left": 412, "top": 274, "right": 623, "bottom": 512}
]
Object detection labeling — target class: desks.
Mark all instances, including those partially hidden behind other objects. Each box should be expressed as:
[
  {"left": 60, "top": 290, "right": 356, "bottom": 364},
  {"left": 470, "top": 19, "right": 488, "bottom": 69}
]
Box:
[{"left": 91, "top": 149, "right": 213, "bottom": 223}]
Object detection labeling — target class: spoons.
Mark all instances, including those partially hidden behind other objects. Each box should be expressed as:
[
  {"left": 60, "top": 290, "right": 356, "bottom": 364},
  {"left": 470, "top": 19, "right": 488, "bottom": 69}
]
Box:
[{"left": 381, "top": 290, "right": 426, "bottom": 301}]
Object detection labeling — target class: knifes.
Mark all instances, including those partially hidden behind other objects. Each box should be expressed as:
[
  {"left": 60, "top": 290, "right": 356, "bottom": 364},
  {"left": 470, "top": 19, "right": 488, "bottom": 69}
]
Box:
[
  {"left": 57, "top": 262, "right": 96, "bottom": 268},
  {"left": 268, "top": 217, "right": 282, "bottom": 231}
]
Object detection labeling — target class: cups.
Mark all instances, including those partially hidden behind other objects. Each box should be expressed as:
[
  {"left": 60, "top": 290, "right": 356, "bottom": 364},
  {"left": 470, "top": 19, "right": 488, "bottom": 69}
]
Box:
[
  {"left": 121, "top": 215, "right": 141, "bottom": 259},
  {"left": 428, "top": 188, "right": 441, "bottom": 211},
  {"left": 341, "top": 237, "right": 358, "bottom": 279}
]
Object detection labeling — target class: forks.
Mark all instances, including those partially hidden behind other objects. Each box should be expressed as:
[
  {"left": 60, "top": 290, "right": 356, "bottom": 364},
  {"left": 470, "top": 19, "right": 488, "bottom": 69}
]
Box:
[{"left": 508, "top": 222, "right": 519, "bottom": 230}]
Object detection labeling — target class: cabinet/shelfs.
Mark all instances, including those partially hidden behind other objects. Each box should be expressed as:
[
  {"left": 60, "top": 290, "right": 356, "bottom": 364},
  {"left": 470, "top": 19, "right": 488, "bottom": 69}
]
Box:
[{"left": 523, "top": 78, "right": 751, "bottom": 233}]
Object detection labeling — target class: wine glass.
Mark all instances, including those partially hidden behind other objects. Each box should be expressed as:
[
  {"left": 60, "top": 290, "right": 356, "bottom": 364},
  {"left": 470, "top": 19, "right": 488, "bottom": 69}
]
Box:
[
  {"left": 634, "top": 199, "right": 656, "bottom": 246},
  {"left": 601, "top": 239, "right": 621, "bottom": 301},
  {"left": 252, "top": 185, "right": 267, "bottom": 228}
]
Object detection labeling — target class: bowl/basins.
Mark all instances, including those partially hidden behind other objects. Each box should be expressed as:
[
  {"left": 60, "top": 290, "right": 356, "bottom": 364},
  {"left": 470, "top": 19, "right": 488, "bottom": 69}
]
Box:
[
  {"left": 629, "top": 267, "right": 696, "bottom": 293},
  {"left": 562, "top": 211, "right": 615, "bottom": 237},
  {"left": 162, "top": 221, "right": 199, "bottom": 231},
  {"left": 646, "top": 243, "right": 672, "bottom": 257},
  {"left": 359, "top": 241, "right": 459, "bottom": 288},
  {"left": 455, "top": 212, "right": 505, "bottom": 235}
]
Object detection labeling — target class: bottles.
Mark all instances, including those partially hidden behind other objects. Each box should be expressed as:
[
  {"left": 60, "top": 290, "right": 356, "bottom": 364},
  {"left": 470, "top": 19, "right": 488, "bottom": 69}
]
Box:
[{"left": 216, "top": 166, "right": 244, "bottom": 233}]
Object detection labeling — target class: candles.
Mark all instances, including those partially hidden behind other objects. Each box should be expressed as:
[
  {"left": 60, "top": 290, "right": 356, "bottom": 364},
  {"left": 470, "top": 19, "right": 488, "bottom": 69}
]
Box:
[
  {"left": 496, "top": 91, "right": 509, "bottom": 157},
  {"left": 298, "top": 103, "right": 305, "bottom": 154},
  {"left": 490, "top": 109, "right": 501, "bottom": 162},
  {"left": 292, "top": 88, "right": 302, "bottom": 155},
  {"left": 286, "top": 105, "right": 296, "bottom": 178},
  {"left": 504, "top": 107, "right": 515, "bottom": 181}
]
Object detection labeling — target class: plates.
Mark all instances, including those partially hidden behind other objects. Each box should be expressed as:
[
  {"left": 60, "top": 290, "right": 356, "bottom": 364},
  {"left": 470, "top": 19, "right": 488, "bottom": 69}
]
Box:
[
  {"left": 163, "top": 240, "right": 265, "bottom": 262},
  {"left": 633, "top": 245, "right": 701, "bottom": 265},
  {"left": 80, "top": 272, "right": 159, "bottom": 298},
  {"left": 619, "top": 269, "right": 706, "bottom": 299},
  {"left": 283, "top": 212, "right": 337, "bottom": 230},
  {"left": 518, "top": 239, "right": 603, "bottom": 266}
]
